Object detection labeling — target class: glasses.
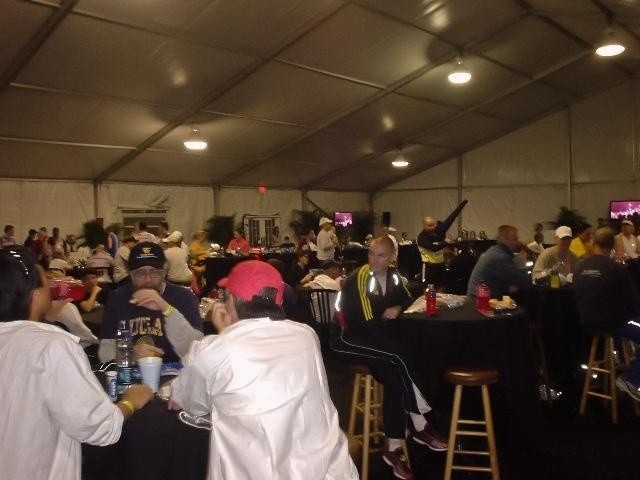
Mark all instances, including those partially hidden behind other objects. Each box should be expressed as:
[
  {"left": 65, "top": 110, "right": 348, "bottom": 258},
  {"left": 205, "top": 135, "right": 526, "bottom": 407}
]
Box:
[{"left": 130, "top": 270, "right": 162, "bottom": 278}]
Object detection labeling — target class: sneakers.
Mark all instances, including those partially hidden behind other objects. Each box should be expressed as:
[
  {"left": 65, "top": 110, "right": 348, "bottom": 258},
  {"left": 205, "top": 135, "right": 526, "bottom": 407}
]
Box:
[
  {"left": 614, "top": 375, "right": 640, "bottom": 402},
  {"left": 381, "top": 446, "right": 413, "bottom": 480},
  {"left": 411, "top": 421, "right": 450, "bottom": 451}
]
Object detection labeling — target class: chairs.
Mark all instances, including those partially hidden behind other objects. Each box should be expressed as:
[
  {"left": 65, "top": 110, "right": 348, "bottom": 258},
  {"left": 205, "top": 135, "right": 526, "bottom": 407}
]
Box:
[
  {"left": 308, "top": 289, "right": 341, "bottom": 361},
  {"left": 44, "top": 263, "right": 209, "bottom": 343}
]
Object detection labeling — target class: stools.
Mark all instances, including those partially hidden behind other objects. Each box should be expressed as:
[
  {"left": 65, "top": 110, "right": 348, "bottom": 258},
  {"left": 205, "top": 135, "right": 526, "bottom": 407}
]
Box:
[
  {"left": 579, "top": 330, "right": 639, "bottom": 422},
  {"left": 344, "top": 363, "right": 411, "bottom": 480},
  {"left": 443, "top": 361, "right": 501, "bottom": 480}
]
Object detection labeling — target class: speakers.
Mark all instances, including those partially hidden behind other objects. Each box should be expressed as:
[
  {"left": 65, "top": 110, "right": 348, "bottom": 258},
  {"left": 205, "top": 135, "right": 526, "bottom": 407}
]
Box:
[{"left": 383, "top": 211, "right": 390, "bottom": 225}]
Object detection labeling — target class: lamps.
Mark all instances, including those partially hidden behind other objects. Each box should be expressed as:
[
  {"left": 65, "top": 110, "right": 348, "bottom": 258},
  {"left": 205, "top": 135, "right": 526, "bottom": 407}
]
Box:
[
  {"left": 183, "top": 124, "right": 208, "bottom": 149},
  {"left": 448, "top": 48, "right": 473, "bottom": 86},
  {"left": 392, "top": 147, "right": 410, "bottom": 167},
  {"left": 593, "top": 13, "right": 627, "bottom": 57}
]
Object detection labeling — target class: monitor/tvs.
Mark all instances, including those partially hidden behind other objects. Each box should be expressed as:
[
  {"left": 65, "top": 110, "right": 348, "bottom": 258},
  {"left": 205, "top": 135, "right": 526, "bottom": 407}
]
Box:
[
  {"left": 609, "top": 199, "right": 640, "bottom": 219},
  {"left": 333, "top": 211, "right": 353, "bottom": 226}
]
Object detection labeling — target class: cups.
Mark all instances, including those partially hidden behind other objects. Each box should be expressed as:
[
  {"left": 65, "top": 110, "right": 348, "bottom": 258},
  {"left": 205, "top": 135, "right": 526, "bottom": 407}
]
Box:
[
  {"left": 470, "top": 231, "right": 475, "bottom": 240},
  {"left": 137, "top": 357, "right": 163, "bottom": 393},
  {"left": 484, "top": 235, "right": 487, "bottom": 240},
  {"left": 480, "top": 231, "right": 485, "bottom": 239}
]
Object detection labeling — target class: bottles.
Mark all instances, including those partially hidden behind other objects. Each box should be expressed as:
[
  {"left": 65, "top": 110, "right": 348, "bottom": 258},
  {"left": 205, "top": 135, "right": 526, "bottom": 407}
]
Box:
[
  {"left": 199, "top": 287, "right": 225, "bottom": 320},
  {"left": 477, "top": 280, "right": 490, "bottom": 313},
  {"left": 116, "top": 331, "right": 134, "bottom": 396},
  {"left": 461, "top": 229, "right": 469, "bottom": 241},
  {"left": 114, "top": 319, "right": 128, "bottom": 377},
  {"left": 550, "top": 270, "right": 560, "bottom": 289},
  {"left": 424, "top": 283, "right": 437, "bottom": 317}
]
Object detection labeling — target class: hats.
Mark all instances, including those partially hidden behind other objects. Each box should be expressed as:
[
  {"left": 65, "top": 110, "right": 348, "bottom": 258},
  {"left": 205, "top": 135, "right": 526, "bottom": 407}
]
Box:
[
  {"left": 554, "top": 226, "right": 573, "bottom": 238},
  {"left": 622, "top": 219, "right": 634, "bottom": 226},
  {"left": 162, "top": 231, "right": 183, "bottom": 243},
  {"left": 49, "top": 258, "right": 68, "bottom": 270},
  {"left": 217, "top": 259, "right": 286, "bottom": 308},
  {"left": 318, "top": 218, "right": 333, "bottom": 227},
  {"left": 127, "top": 241, "right": 166, "bottom": 270}
]
